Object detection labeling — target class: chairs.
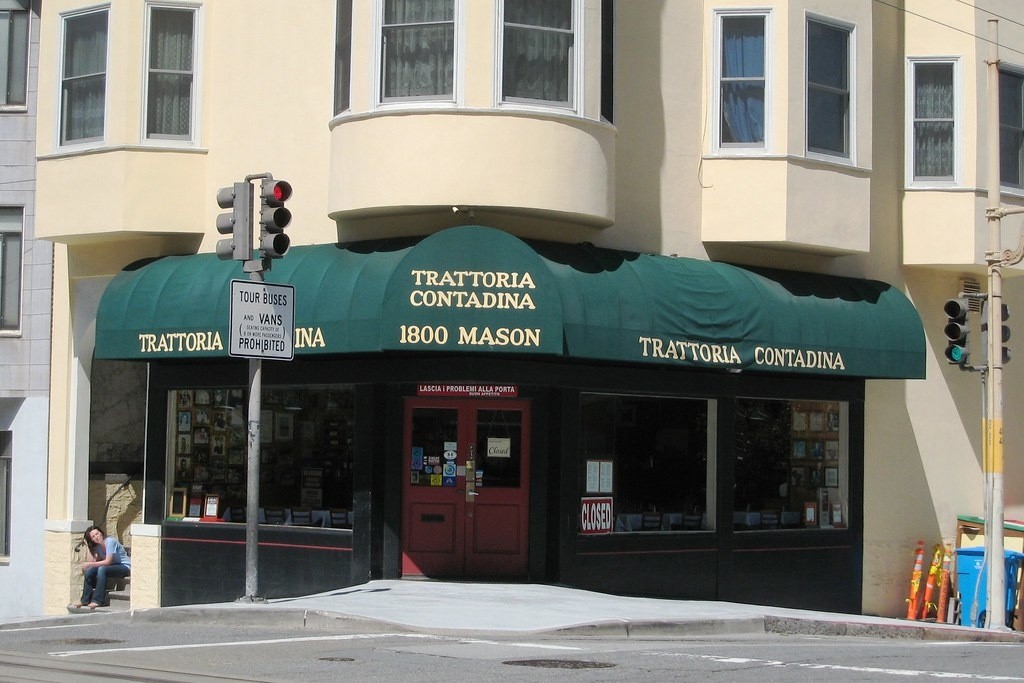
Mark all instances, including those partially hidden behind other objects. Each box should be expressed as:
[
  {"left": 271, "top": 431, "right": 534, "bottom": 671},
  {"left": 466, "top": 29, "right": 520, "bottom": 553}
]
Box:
[
  {"left": 759, "top": 510, "right": 783, "bottom": 529},
  {"left": 681, "top": 510, "right": 703, "bottom": 530},
  {"left": 642, "top": 512, "right": 664, "bottom": 531}
]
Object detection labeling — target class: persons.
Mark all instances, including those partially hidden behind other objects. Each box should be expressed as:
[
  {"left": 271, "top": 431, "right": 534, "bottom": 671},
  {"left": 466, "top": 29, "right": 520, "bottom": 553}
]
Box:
[
  {"left": 177, "top": 388, "right": 230, "bottom": 483},
  {"left": 73, "top": 525, "right": 131, "bottom": 608}
]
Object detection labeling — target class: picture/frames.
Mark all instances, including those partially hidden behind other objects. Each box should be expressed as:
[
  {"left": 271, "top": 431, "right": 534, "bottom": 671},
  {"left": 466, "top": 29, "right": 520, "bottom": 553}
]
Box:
[
  {"left": 178, "top": 434, "right": 191, "bottom": 454},
  {"left": 192, "top": 446, "right": 211, "bottom": 464},
  {"left": 211, "top": 389, "right": 245, "bottom": 502},
  {"left": 193, "top": 405, "right": 211, "bottom": 428},
  {"left": 195, "top": 388, "right": 213, "bottom": 404},
  {"left": 275, "top": 412, "right": 294, "bottom": 441},
  {"left": 260, "top": 409, "right": 273, "bottom": 443},
  {"left": 193, "top": 463, "right": 211, "bottom": 482},
  {"left": 191, "top": 427, "right": 211, "bottom": 447},
  {"left": 264, "top": 390, "right": 280, "bottom": 406},
  {"left": 261, "top": 447, "right": 277, "bottom": 465},
  {"left": 176, "top": 456, "right": 193, "bottom": 481},
  {"left": 178, "top": 411, "right": 192, "bottom": 433},
  {"left": 203, "top": 493, "right": 219, "bottom": 518},
  {"left": 170, "top": 488, "right": 187, "bottom": 518},
  {"left": 177, "top": 390, "right": 194, "bottom": 409},
  {"left": 616, "top": 404, "right": 636, "bottom": 429},
  {"left": 298, "top": 421, "right": 315, "bottom": 440}
]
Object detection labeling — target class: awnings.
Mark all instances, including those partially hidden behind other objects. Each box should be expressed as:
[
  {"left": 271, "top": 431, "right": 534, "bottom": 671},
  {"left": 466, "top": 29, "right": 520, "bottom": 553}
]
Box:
[{"left": 93, "top": 225, "right": 927, "bottom": 379}]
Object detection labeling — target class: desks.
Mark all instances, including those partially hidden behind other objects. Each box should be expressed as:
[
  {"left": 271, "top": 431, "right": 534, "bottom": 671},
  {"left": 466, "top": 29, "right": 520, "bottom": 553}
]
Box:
[
  {"left": 951, "top": 515, "right": 1024, "bottom": 631},
  {"left": 614, "top": 511, "right": 802, "bottom": 532}
]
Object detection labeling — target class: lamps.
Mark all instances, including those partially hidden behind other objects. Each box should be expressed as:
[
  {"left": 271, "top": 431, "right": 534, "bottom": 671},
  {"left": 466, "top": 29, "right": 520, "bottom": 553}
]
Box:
[{"left": 285, "top": 401, "right": 303, "bottom": 410}]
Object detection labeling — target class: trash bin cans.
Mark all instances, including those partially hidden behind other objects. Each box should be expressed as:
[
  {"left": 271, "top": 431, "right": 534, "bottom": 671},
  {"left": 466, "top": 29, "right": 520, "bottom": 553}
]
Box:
[{"left": 955, "top": 546, "right": 1024, "bottom": 628}]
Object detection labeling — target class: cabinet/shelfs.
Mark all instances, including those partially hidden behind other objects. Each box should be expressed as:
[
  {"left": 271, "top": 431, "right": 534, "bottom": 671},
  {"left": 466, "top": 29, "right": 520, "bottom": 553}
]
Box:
[
  {"left": 321, "top": 416, "right": 354, "bottom": 472},
  {"left": 786, "top": 400, "right": 839, "bottom": 511}
]
